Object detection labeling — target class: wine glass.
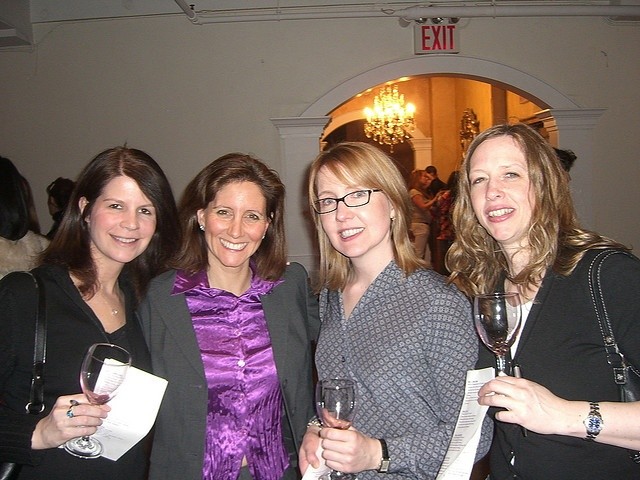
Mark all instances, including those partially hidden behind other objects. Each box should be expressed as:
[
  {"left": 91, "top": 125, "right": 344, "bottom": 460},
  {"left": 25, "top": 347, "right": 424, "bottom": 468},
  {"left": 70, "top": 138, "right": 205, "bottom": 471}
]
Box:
[
  {"left": 62, "top": 343, "right": 132, "bottom": 459},
  {"left": 473, "top": 292, "right": 522, "bottom": 398},
  {"left": 315, "top": 379, "right": 358, "bottom": 480}
]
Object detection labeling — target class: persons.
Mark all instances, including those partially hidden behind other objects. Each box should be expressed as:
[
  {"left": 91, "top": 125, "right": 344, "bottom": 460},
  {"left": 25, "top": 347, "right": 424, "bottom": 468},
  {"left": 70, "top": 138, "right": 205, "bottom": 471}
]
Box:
[
  {"left": 135, "top": 152, "right": 321, "bottom": 480},
  {"left": 1, "top": 146, "right": 182, "bottom": 480},
  {"left": 443, "top": 124, "right": 639, "bottom": 480},
  {"left": 407, "top": 170, "right": 443, "bottom": 260},
  {"left": 528, "top": 121, "right": 549, "bottom": 143},
  {"left": 436, "top": 170, "right": 462, "bottom": 275},
  {"left": 0, "top": 157, "right": 53, "bottom": 278},
  {"left": 46, "top": 176, "right": 75, "bottom": 238},
  {"left": 425, "top": 166, "right": 447, "bottom": 196},
  {"left": 551, "top": 148, "right": 577, "bottom": 183},
  {"left": 297, "top": 141, "right": 495, "bottom": 480}
]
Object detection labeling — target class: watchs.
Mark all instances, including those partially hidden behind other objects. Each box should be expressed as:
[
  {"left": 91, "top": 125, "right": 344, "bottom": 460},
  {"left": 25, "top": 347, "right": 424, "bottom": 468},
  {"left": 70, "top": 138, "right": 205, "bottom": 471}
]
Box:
[
  {"left": 584, "top": 402, "right": 604, "bottom": 441},
  {"left": 376, "top": 438, "right": 390, "bottom": 473}
]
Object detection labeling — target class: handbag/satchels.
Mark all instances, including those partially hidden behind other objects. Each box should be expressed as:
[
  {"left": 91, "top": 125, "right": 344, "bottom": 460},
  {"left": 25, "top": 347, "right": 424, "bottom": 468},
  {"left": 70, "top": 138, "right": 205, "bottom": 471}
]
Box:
[
  {"left": 587, "top": 245, "right": 640, "bottom": 462},
  {"left": 1, "top": 270, "right": 49, "bottom": 480}
]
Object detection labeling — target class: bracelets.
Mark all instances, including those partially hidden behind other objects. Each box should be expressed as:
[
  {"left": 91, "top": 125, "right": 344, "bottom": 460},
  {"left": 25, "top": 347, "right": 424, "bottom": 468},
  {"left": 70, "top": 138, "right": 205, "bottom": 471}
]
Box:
[{"left": 306, "top": 420, "right": 320, "bottom": 428}]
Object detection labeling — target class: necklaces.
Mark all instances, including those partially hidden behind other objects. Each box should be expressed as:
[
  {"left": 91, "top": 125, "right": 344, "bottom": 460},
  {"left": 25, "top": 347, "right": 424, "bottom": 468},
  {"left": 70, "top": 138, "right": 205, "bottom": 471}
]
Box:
[{"left": 98, "top": 288, "right": 125, "bottom": 315}]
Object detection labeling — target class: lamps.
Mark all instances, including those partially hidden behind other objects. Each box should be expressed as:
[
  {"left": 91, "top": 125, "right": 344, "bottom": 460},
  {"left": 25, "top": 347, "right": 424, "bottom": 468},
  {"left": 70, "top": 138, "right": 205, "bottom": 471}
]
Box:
[{"left": 362, "top": 84, "right": 417, "bottom": 152}]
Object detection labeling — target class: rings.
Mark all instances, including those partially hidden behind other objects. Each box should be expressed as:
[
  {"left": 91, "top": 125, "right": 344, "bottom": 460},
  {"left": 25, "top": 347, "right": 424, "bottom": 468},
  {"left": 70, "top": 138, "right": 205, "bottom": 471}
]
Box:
[{"left": 67, "top": 405, "right": 75, "bottom": 418}]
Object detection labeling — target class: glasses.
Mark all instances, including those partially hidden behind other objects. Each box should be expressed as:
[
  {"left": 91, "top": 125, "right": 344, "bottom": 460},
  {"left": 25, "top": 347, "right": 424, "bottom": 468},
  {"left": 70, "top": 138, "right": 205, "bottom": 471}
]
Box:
[{"left": 310, "top": 188, "right": 382, "bottom": 214}]
